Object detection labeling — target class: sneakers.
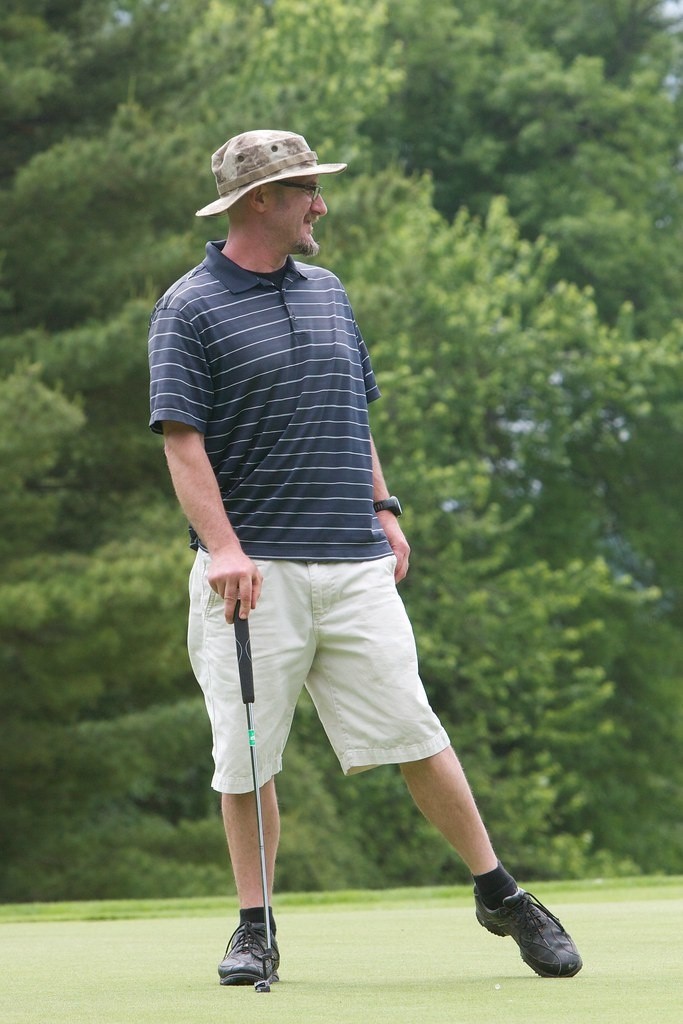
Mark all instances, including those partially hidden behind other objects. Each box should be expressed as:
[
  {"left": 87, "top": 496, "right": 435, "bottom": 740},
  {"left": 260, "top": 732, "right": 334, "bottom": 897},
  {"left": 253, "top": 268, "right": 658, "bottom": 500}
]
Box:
[
  {"left": 476, "top": 869, "right": 584, "bottom": 979},
  {"left": 218, "top": 921, "right": 281, "bottom": 987}
]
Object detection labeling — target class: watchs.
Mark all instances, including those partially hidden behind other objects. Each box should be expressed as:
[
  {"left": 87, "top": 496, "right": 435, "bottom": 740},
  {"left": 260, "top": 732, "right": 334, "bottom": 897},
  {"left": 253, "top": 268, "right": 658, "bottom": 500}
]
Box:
[{"left": 373, "top": 495, "right": 403, "bottom": 518}]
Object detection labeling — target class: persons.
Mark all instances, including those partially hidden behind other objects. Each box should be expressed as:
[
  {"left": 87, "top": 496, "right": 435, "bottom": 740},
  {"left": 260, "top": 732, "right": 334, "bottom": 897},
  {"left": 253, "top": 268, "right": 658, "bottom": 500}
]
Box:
[{"left": 147, "top": 130, "right": 583, "bottom": 986}]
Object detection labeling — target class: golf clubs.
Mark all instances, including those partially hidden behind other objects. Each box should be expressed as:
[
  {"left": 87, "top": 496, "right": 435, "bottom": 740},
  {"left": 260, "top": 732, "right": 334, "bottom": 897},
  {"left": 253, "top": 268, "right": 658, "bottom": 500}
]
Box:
[{"left": 233, "top": 587, "right": 271, "bottom": 993}]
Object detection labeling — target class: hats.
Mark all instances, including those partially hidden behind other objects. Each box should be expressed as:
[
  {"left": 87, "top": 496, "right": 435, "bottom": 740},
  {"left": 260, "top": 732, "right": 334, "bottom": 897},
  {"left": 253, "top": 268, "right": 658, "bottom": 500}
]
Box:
[{"left": 194, "top": 129, "right": 347, "bottom": 217}]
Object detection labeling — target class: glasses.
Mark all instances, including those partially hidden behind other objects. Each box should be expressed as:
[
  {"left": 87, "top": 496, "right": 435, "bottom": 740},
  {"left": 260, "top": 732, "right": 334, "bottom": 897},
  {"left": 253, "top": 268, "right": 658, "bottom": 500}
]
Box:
[{"left": 274, "top": 180, "right": 322, "bottom": 201}]
